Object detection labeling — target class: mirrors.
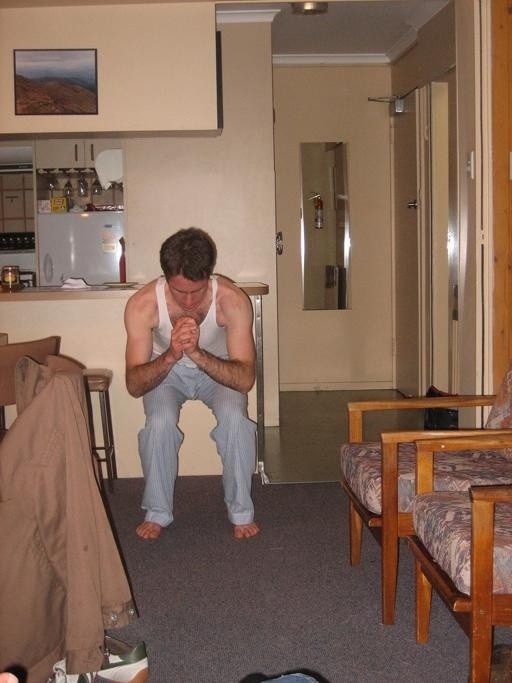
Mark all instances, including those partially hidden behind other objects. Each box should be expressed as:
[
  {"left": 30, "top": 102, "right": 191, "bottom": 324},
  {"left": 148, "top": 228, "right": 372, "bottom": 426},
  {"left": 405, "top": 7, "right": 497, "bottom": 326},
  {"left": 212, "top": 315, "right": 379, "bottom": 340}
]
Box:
[{"left": 300, "top": 141, "right": 352, "bottom": 311}]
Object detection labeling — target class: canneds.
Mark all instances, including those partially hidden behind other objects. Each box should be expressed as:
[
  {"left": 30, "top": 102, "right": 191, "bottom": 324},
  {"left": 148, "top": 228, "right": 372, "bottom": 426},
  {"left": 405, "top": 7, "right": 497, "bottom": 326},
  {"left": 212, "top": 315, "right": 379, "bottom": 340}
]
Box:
[{"left": 2, "top": 266, "right": 20, "bottom": 293}]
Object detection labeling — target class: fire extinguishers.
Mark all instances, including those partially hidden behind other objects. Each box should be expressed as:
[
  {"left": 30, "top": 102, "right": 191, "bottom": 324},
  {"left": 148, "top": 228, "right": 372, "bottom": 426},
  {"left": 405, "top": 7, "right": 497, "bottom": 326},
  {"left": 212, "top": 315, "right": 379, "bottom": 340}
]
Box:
[{"left": 308, "top": 192, "right": 324, "bottom": 229}]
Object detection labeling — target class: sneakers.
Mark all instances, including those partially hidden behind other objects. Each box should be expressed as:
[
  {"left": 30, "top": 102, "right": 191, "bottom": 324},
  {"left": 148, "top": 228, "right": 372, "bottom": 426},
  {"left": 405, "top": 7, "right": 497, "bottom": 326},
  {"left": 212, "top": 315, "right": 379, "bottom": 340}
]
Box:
[{"left": 46, "top": 635, "right": 148, "bottom": 683}]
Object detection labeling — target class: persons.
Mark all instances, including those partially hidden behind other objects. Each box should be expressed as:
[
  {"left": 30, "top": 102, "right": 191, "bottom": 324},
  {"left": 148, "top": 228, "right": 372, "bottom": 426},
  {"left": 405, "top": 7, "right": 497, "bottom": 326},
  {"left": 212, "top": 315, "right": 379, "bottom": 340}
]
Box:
[{"left": 124, "top": 228, "right": 261, "bottom": 540}]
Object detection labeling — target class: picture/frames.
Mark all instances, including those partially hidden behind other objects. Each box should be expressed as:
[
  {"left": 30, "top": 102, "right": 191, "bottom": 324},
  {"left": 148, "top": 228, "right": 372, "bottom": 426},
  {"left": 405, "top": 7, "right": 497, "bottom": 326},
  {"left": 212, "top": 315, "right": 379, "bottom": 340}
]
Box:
[{"left": 12, "top": 47, "right": 99, "bottom": 116}]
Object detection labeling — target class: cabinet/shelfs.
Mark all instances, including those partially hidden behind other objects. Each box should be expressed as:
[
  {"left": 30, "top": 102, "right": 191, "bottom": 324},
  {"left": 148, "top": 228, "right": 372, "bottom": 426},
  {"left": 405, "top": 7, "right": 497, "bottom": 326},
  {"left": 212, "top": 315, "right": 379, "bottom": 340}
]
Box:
[{"left": 32, "top": 137, "right": 126, "bottom": 295}]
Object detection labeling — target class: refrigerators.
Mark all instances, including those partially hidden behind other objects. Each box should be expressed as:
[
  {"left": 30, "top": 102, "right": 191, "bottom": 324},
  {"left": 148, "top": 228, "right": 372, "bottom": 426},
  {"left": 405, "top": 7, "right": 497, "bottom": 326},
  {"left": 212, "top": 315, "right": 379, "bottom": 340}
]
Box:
[
  {"left": 101, "top": 282, "right": 137, "bottom": 289},
  {"left": 36, "top": 210, "right": 124, "bottom": 288}
]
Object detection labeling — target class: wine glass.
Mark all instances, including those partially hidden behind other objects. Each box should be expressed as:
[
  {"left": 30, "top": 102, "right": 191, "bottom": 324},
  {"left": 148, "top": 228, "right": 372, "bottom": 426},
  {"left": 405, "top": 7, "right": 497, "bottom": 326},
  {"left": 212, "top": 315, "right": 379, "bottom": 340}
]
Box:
[{"left": 41, "top": 171, "right": 103, "bottom": 198}]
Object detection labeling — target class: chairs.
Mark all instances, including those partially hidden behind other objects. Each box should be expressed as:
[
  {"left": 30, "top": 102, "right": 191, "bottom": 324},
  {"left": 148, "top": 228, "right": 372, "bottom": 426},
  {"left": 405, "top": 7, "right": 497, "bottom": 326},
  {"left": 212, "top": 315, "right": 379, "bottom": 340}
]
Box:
[
  {"left": 334, "top": 367, "right": 511, "bottom": 682},
  {"left": 0, "top": 332, "right": 120, "bottom": 498}
]
